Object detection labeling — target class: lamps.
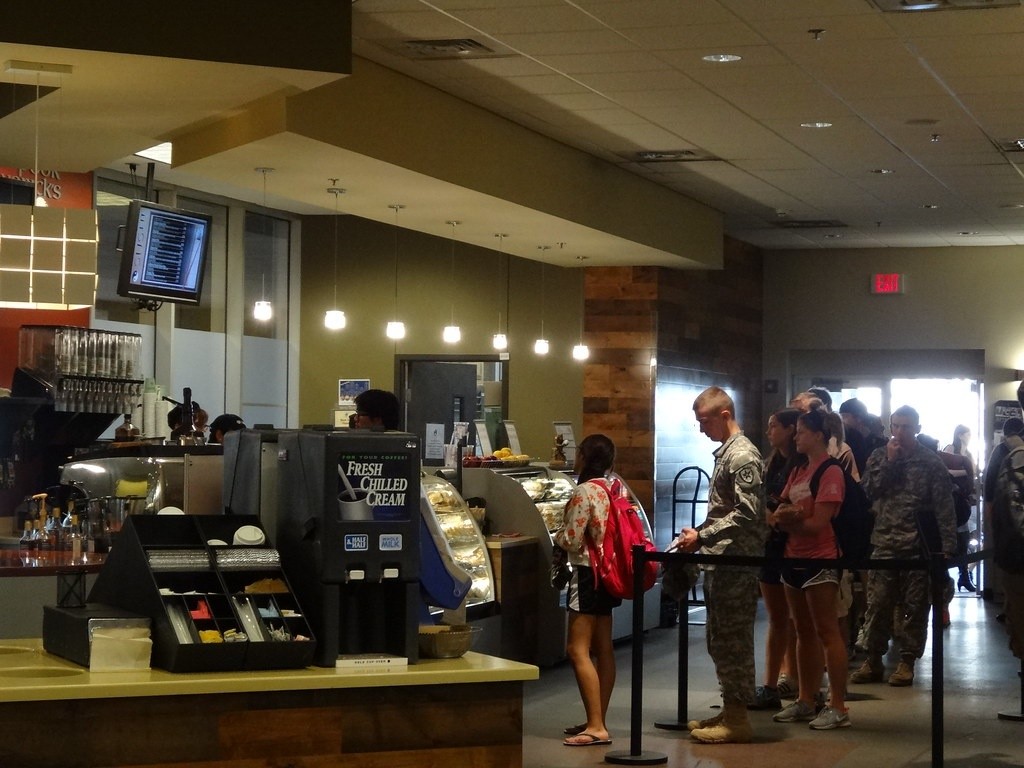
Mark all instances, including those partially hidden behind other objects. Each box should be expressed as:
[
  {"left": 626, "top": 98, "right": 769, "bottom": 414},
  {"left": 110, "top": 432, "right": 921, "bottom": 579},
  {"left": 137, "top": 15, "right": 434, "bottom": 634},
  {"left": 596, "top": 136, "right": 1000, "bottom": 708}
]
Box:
[
  {"left": 535, "top": 246, "right": 549, "bottom": 355},
  {"left": 573, "top": 256, "right": 589, "bottom": 361},
  {"left": 443, "top": 220, "right": 461, "bottom": 343},
  {"left": 323, "top": 188, "right": 346, "bottom": 329},
  {"left": 254, "top": 166, "right": 275, "bottom": 322},
  {"left": 493, "top": 233, "right": 508, "bottom": 349},
  {"left": 387, "top": 206, "right": 406, "bottom": 340}
]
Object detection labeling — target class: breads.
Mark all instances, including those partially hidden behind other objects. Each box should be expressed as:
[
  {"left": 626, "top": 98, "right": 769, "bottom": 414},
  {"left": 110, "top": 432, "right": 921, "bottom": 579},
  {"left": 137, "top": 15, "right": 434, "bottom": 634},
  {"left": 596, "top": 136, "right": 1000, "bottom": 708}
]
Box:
[
  {"left": 519, "top": 481, "right": 570, "bottom": 532},
  {"left": 427, "top": 491, "right": 492, "bottom": 599}
]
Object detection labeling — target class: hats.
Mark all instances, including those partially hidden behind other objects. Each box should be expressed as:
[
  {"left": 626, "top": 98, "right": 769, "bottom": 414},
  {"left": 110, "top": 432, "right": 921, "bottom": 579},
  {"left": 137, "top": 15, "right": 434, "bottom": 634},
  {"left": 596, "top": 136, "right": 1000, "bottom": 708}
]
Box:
[
  {"left": 840, "top": 398, "right": 868, "bottom": 413},
  {"left": 168, "top": 401, "right": 200, "bottom": 430},
  {"left": 211, "top": 414, "right": 246, "bottom": 435}
]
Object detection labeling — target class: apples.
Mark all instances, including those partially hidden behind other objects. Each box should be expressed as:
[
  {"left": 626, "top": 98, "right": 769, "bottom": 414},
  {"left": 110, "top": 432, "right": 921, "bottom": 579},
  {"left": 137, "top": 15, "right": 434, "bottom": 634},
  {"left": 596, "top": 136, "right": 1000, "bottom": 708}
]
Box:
[{"left": 462, "top": 454, "right": 505, "bottom": 467}]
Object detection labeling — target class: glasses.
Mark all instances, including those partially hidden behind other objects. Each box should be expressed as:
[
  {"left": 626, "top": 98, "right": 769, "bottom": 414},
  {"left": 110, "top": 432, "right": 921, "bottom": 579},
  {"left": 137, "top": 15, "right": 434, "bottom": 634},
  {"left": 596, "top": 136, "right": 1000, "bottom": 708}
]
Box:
[
  {"left": 195, "top": 423, "right": 209, "bottom": 433},
  {"left": 354, "top": 411, "right": 369, "bottom": 419}
]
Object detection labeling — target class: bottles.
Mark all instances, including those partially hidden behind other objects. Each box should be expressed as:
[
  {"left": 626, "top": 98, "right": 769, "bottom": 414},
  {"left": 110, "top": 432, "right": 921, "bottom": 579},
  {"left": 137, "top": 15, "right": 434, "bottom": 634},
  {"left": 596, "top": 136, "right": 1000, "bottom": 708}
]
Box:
[
  {"left": 19, "top": 508, "right": 96, "bottom": 566},
  {"left": 115, "top": 413, "right": 140, "bottom": 442}
]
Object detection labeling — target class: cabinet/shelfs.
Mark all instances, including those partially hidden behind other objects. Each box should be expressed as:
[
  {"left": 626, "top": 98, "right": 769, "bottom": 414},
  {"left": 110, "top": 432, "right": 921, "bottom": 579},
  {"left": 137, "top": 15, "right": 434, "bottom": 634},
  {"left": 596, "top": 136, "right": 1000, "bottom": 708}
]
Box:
[
  {"left": 461, "top": 456, "right": 661, "bottom": 665},
  {"left": 420, "top": 475, "right": 501, "bottom": 659},
  {"left": 88, "top": 514, "right": 318, "bottom": 672}
]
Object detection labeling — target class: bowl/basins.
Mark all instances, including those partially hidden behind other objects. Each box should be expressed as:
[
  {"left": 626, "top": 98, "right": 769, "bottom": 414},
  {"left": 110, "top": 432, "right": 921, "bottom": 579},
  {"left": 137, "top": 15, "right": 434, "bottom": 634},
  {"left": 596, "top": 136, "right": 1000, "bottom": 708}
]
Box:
[
  {"left": 461, "top": 456, "right": 535, "bottom": 468},
  {"left": 419, "top": 625, "right": 482, "bottom": 658}
]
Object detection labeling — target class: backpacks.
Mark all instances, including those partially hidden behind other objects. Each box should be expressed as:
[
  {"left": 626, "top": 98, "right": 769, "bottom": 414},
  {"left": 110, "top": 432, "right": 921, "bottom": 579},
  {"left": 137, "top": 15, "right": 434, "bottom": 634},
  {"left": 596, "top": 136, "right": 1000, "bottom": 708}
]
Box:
[
  {"left": 810, "top": 455, "right": 867, "bottom": 564},
  {"left": 993, "top": 437, "right": 1024, "bottom": 574},
  {"left": 585, "top": 479, "right": 658, "bottom": 601}
]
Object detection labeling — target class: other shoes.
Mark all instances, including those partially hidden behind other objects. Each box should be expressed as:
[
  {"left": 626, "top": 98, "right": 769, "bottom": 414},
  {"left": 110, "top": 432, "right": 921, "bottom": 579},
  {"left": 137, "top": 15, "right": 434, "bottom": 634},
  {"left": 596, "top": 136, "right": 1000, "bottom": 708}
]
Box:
[
  {"left": 773, "top": 674, "right": 799, "bottom": 699},
  {"left": 750, "top": 687, "right": 783, "bottom": 711},
  {"left": 850, "top": 654, "right": 884, "bottom": 683},
  {"left": 888, "top": 655, "right": 914, "bottom": 686}
]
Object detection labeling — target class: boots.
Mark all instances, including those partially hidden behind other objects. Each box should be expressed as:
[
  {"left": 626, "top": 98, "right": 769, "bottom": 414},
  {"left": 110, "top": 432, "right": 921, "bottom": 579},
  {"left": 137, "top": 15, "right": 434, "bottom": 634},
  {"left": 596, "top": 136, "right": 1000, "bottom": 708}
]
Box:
[
  {"left": 691, "top": 702, "right": 753, "bottom": 742},
  {"left": 688, "top": 706, "right": 723, "bottom": 730}
]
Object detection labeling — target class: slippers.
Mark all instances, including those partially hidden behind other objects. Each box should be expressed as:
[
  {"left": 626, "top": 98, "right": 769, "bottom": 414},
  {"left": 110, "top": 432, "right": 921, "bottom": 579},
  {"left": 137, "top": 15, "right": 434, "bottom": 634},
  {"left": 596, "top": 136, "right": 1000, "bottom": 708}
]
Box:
[
  {"left": 563, "top": 727, "right": 584, "bottom": 732},
  {"left": 563, "top": 733, "right": 612, "bottom": 746}
]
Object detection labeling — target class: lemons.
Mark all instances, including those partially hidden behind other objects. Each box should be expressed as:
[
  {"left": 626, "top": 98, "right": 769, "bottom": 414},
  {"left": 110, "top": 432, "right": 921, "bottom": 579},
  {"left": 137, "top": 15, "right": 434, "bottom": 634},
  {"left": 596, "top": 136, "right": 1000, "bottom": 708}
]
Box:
[{"left": 492, "top": 448, "right": 530, "bottom": 467}]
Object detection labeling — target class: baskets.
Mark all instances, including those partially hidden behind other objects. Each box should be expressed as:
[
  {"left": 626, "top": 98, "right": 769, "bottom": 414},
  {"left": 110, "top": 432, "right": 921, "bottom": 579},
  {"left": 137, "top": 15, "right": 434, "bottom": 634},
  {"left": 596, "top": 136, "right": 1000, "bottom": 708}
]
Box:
[{"left": 419, "top": 624, "right": 482, "bottom": 658}]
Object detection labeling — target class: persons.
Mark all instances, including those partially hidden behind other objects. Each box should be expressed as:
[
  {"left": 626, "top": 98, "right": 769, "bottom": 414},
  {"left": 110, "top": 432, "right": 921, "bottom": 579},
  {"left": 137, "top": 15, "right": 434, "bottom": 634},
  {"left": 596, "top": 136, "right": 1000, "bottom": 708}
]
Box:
[
  {"left": 555, "top": 434, "right": 624, "bottom": 746},
  {"left": 982, "top": 379, "right": 1024, "bottom": 661},
  {"left": 748, "top": 387, "right": 978, "bottom": 731},
  {"left": 354, "top": 389, "right": 400, "bottom": 431},
  {"left": 676, "top": 387, "right": 768, "bottom": 742},
  {"left": 168, "top": 401, "right": 208, "bottom": 433},
  {"left": 208, "top": 413, "right": 247, "bottom": 443}
]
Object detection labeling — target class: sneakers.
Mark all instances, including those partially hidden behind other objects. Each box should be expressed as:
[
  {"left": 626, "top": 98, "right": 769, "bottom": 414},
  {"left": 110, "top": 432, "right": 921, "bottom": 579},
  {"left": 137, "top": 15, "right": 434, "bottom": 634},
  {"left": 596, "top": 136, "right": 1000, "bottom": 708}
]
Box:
[
  {"left": 772, "top": 701, "right": 816, "bottom": 722},
  {"left": 809, "top": 705, "right": 851, "bottom": 729}
]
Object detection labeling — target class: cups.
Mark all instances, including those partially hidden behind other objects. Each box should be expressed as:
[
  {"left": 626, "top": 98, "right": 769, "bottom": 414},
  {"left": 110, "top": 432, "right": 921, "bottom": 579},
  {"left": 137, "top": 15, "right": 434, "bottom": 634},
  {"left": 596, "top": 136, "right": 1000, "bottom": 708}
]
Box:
[
  {"left": 337, "top": 488, "right": 376, "bottom": 521},
  {"left": 132, "top": 377, "right": 173, "bottom": 440},
  {"left": 443, "top": 422, "right": 473, "bottom": 468}
]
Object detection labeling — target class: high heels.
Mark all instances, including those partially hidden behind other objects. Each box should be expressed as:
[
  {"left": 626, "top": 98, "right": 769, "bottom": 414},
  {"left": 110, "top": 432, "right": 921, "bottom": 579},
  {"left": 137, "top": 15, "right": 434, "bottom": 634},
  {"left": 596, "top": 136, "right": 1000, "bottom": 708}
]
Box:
[{"left": 958, "top": 574, "right": 977, "bottom": 592}]
